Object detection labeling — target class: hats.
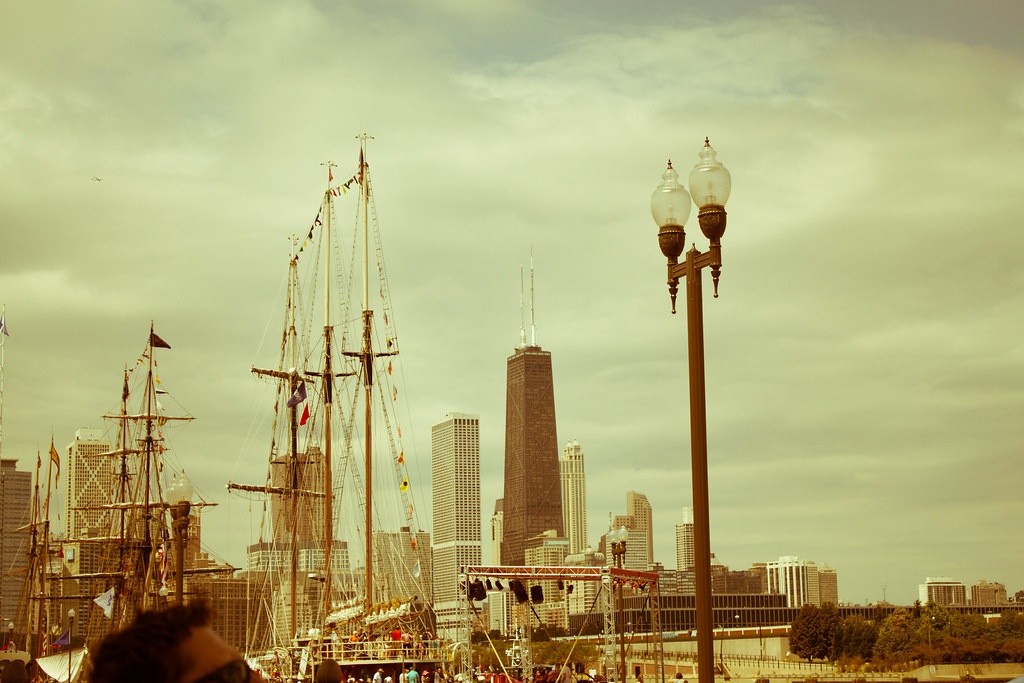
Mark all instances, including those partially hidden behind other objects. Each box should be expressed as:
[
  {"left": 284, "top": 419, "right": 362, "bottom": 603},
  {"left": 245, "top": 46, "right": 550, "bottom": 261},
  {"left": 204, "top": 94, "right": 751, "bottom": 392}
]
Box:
[{"left": 377, "top": 668, "right": 385, "bottom": 673}]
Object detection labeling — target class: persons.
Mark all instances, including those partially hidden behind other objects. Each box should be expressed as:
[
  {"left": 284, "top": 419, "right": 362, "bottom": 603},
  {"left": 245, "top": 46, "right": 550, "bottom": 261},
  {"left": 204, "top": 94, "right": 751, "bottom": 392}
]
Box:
[
  {"left": 670, "top": 672, "right": 688, "bottom": 683},
  {"left": 637, "top": 674, "right": 644, "bottom": 683},
  {"left": 268, "top": 648, "right": 301, "bottom": 683},
  {"left": 7, "top": 637, "right": 16, "bottom": 650},
  {"left": 78, "top": 601, "right": 262, "bottom": 683},
  {"left": 385, "top": 626, "right": 432, "bottom": 659},
  {"left": 289, "top": 627, "right": 364, "bottom": 657},
  {"left": 316, "top": 659, "right": 454, "bottom": 683},
  {"left": 0, "top": 658, "right": 44, "bottom": 683}
]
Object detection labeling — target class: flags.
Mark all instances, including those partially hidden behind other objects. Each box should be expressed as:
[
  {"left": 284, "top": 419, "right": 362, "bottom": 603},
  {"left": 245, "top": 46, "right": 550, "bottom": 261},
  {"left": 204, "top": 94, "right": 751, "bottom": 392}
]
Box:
[
  {"left": 300, "top": 404, "right": 310, "bottom": 425},
  {"left": 52, "top": 446, "right": 60, "bottom": 488},
  {"left": 397, "top": 426, "right": 420, "bottom": 578},
  {"left": 329, "top": 175, "right": 358, "bottom": 197},
  {"left": 156, "top": 541, "right": 168, "bottom": 596},
  {"left": 287, "top": 381, "right": 307, "bottom": 408},
  {"left": 293, "top": 207, "right": 322, "bottom": 260},
  {"left": 153, "top": 333, "right": 171, "bottom": 348},
  {"left": 122, "top": 381, "right": 128, "bottom": 402},
  {"left": 384, "top": 313, "right": 398, "bottom": 402},
  {"left": 94, "top": 586, "right": 115, "bottom": 618},
  {"left": 49, "top": 630, "right": 69, "bottom": 648}
]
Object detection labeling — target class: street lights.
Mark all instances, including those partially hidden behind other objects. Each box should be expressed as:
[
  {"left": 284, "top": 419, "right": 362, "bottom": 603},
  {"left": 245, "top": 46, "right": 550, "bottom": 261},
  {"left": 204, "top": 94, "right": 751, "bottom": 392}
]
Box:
[
  {"left": 719, "top": 615, "right": 740, "bottom": 673},
  {"left": 626, "top": 622, "right": 637, "bottom": 678},
  {"left": 65, "top": 608, "right": 77, "bottom": 683},
  {"left": 928, "top": 612, "right": 938, "bottom": 676},
  {"left": 651, "top": 134, "right": 733, "bottom": 683},
  {"left": 7, "top": 621, "right": 15, "bottom": 642},
  {"left": 606, "top": 525, "right": 633, "bottom": 683},
  {"left": 164, "top": 470, "right": 195, "bottom": 607}
]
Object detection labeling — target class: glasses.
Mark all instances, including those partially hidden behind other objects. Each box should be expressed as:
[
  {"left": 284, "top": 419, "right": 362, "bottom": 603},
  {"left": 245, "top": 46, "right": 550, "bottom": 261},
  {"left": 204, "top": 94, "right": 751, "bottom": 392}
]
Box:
[{"left": 192, "top": 657, "right": 252, "bottom": 683}]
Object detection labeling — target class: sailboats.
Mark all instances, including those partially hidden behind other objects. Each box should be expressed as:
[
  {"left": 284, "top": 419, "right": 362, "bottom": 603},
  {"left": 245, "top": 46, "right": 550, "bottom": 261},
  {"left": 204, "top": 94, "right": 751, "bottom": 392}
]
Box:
[{"left": 235, "top": 130, "right": 465, "bottom": 682}]
